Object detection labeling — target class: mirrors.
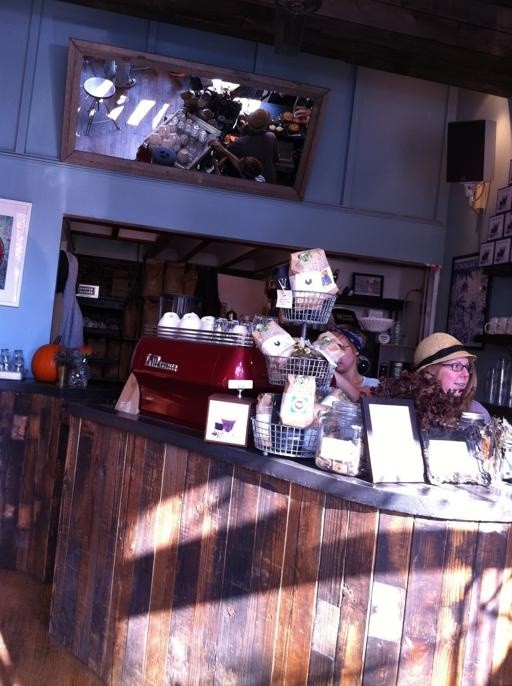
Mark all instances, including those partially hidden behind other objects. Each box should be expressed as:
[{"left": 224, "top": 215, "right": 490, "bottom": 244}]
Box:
[{"left": 59, "top": 37, "right": 333, "bottom": 202}]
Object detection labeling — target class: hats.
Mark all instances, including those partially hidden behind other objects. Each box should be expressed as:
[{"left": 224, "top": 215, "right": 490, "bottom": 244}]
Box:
[
  {"left": 249, "top": 108, "right": 271, "bottom": 132},
  {"left": 413, "top": 332, "right": 477, "bottom": 373}
]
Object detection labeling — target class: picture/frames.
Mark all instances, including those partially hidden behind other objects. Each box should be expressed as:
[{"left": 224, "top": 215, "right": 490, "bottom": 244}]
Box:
[
  {"left": 351, "top": 271, "right": 385, "bottom": 299},
  {"left": 445, "top": 253, "right": 493, "bottom": 352},
  {"left": 0, "top": 196, "right": 33, "bottom": 309}
]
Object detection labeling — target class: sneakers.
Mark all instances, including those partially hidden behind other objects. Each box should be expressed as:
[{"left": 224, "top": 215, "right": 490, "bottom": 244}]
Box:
[{"left": 105, "top": 76, "right": 136, "bottom": 88}]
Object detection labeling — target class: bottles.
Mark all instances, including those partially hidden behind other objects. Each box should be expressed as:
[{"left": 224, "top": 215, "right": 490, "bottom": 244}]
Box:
[
  {"left": 458, "top": 412, "right": 493, "bottom": 490},
  {"left": 484, "top": 358, "right": 512, "bottom": 408},
  {"left": 316, "top": 399, "right": 366, "bottom": 478},
  {"left": 68, "top": 353, "right": 92, "bottom": 388}
]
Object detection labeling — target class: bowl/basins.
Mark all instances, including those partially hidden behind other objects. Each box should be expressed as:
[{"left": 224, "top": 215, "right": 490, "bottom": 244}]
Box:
[{"left": 361, "top": 317, "right": 393, "bottom": 334}]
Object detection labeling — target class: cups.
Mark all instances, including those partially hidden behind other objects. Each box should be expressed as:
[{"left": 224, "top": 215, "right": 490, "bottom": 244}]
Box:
[
  {"left": 173, "top": 118, "right": 207, "bottom": 145},
  {"left": 159, "top": 309, "right": 279, "bottom": 350},
  {"left": 484, "top": 316, "right": 512, "bottom": 336},
  {"left": 0, "top": 348, "right": 24, "bottom": 374}
]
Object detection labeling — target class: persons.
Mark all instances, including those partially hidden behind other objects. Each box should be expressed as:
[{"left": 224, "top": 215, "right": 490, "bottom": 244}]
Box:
[
  {"left": 219, "top": 110, "right": 279, "bottom": 184},
  {"left": 312, "top": 324, "right": 381, "bottom": 402},
  {"left": 210, "top": 142, "right": 266, "bottom": 183},
  {"left": 413, "top": 332, "right": 495, "bottom": 459}
]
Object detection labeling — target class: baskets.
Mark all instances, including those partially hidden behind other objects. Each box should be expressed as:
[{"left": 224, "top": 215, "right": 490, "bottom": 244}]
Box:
[
  {"left": 250, "top": 415, "right": 319, "bottom": 457},
  {"left": 280, "top": 290, "right": 337, "bottom": 324},
  {"left": 264, "top": 356, "right": 337, "bottom": 390}
]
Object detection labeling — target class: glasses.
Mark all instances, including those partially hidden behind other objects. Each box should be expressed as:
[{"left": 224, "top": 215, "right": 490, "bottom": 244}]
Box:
[
  {"left": 340, "top": 346, "right": 351, "bottom": 350},
  {"left": 442, "top": 363, "right": 473, "bottom": 374}
]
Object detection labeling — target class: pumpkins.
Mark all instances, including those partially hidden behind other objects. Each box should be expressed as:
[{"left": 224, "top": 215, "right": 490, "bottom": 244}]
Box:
[{"left": 31, "top": 336, "right": 67, "bottom": 381}]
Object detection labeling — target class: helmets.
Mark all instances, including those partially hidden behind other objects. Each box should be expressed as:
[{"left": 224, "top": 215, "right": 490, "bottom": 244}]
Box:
[{"left": 153, "top": 146, "right": 177, "bottom": 162}]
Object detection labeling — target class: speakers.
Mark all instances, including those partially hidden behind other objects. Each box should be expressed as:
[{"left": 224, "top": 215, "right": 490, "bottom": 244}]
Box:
[{"left": 445, "top": 120, "right": 495, "bottom": 184}]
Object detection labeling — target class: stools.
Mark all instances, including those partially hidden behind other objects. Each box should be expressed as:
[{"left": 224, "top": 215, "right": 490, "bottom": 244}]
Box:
[{"left": 82, "top": 77, "right": 120, "bottom": 136}]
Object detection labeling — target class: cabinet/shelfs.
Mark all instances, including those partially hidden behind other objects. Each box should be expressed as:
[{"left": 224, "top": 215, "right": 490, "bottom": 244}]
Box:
[{"left": 74, "top": 296, "right": 127, "bottom": 385}]
